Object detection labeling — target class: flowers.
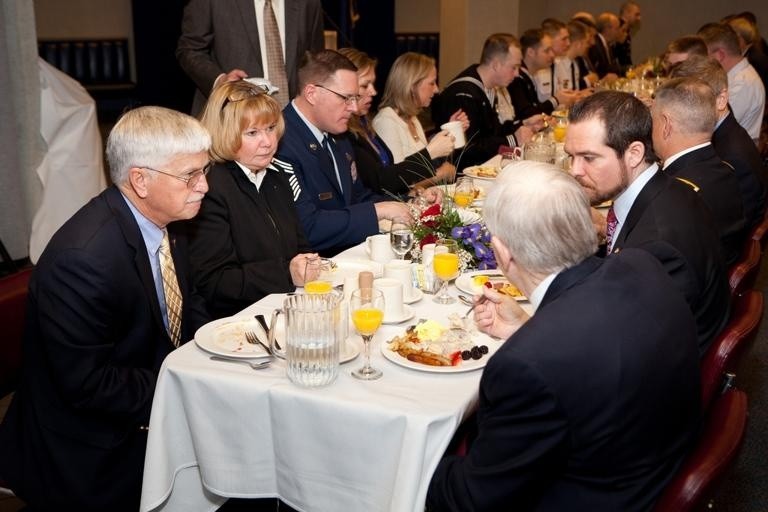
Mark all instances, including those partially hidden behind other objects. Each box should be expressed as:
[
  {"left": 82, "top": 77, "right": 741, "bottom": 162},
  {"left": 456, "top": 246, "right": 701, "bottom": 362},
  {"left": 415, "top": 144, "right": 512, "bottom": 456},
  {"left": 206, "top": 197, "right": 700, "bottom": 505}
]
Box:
[{"left": 381, "top": 128, "right": 497, "bottom": 271}]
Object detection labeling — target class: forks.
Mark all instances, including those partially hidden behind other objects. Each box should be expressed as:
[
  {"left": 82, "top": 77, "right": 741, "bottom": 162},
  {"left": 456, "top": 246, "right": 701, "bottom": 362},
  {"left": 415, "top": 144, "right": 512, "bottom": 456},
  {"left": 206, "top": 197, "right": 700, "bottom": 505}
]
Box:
[{"left": 209, "top": 331, "right": 271, "bottom": 370}]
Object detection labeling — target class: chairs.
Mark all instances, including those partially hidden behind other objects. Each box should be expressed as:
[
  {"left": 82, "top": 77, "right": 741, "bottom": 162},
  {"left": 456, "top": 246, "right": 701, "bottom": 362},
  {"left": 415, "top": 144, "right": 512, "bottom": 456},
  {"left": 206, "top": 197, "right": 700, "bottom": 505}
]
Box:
[
  {"left": 751, "top": 207, "right": 768, "bottom": 241},
  {"left": 691, "top": 292, "right": 762, "bottom": 412},
  {"left": 728, "top": 241, "right": 763, "bottom": 294},
  {"left": 1, "top": 268, "right": 33, "bottom": 510},
  {"left": 658, "top": 388, "right": 747, "bottom": 511},
  {"left": 754, "top": 123, "right": 767, "bottom": 158}
]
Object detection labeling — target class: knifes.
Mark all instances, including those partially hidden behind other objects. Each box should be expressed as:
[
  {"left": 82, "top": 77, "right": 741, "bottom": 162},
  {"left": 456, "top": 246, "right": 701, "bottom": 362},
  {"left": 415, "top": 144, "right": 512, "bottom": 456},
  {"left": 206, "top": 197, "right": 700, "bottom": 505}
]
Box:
[{"left": 255, "top": 314, "right": 282, "bottom": 351}]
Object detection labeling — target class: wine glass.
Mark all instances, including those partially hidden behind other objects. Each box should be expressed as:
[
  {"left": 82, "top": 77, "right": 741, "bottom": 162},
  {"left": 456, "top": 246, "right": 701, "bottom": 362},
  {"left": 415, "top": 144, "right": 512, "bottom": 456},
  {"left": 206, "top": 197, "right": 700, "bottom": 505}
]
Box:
[
  {"left": 613, "top": 66, "right": 660, "bottom": 96},
  {"left": 349, "top": 288, "right": 385, "bottom": 381},
  {"left": 434, "top": 238, "right": 458, "bottom": 303}
]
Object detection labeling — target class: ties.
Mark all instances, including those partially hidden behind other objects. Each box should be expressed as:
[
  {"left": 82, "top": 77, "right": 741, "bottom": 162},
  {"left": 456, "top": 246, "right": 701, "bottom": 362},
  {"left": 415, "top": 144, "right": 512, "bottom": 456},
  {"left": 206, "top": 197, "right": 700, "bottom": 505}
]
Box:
[
  {"left": 264, "top": 0, "right": 290, "bottom": 113},
  {"left": 607, "top": 202, "right": 618, "bottom": 257},
  {"left": 159, "top": 232, "right": 183, "bottom": 348}
]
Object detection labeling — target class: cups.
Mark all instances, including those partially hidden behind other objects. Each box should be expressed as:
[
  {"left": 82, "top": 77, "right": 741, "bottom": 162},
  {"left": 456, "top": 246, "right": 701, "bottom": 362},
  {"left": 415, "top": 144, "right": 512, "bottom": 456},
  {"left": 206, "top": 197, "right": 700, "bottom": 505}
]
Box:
[
  {"left": 267, "top": 293, "right": 344, "bottom": 388},
  {"left": 364, "top": 232, "right": 398, "bottom": 262},
  {"left": 374, "top": 278, "right": 405, "bottom": 320},
  {"left": 422, "top": 242, "right": 449, "bottom": 296},
  {"left": 304, "top": 258, "right": 340, "bottom": 298},
  {"left": 383, "top": 260, "right": 412, "bottom": 300}
]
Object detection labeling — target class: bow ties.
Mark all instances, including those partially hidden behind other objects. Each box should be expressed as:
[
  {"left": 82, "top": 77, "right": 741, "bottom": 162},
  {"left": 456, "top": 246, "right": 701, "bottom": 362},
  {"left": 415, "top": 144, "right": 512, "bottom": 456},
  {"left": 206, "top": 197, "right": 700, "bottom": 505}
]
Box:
[{"left": 322, "top": 133, "right": 333, "bottom": 163}]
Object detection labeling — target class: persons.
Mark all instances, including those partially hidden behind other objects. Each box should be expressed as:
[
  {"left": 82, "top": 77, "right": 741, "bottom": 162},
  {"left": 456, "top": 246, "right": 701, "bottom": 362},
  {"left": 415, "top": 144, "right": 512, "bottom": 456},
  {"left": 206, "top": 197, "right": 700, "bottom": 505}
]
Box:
[
  {"left": 434, "top": 32, "right": 549, "bottom": 172},
  {"left": 270, "top": 50, "right": 414, "bottom": 255},
  {"left": 335, "top": 49, "right": 470, "bottom": 198},
  {"left": 642, "top": 11, "right": 768, "bottom": 183},
  {"left": 504, "top": 29, "right": 577, "bottom": 121},
  {"left": 170, "top": 0, "right": 326, "bottom": 131},
  {"left": 561, "top": 92, "right": 733, "bottom": 359},
  {"left": 554, "top": 2, "right": 642, "bottom": 91},
  {"left": 370, "top": 50, "right": 469, "bottom": 165},
  {"left": 0, "top": 106, "right": 249, "bottom": 512},
  {"left": 535, "top": 18, "right": 588, "bottom": 110},
  {"left": 174, "top": 75, "right": 307, "bottom": 328},
  {"left": 408, "top": 164, "right": 702, "bottom": 511}
]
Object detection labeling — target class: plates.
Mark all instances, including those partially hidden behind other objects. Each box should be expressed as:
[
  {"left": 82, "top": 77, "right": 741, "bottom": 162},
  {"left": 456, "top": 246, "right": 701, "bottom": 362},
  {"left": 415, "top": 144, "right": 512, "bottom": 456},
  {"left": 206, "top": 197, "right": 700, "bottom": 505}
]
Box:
[
  {"left": 318, "top": 257, "right": 385, "bottom": 287},
  {"left": 193, "top": 312, "right": 286, "bottom": 360},
  {"left": 454, "top": 270, "right": 528, "bottom": 302},
  {"left": 272, "top": 312, "right": 361, "bottom": 366},
  {"left": 405, "top": 288, "right": 426, "bottom": 305},
  {"left": 364, "top": 115, "right": 569, "bottom": 271},
  {"left": 381, "top": 326, "right": 500, "bottom": 373},
  {"left": 363, "top": 302, "right": 417, "bottom": 325}
]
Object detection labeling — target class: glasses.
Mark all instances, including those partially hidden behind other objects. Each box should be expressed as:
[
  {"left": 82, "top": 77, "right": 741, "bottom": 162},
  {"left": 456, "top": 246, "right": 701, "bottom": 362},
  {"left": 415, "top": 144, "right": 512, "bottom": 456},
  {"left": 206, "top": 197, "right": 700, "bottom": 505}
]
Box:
[
  {"left": 140, "top": 162, "right": 213, "bottom": 188},
  {"left": 222, "top": 84, "right": 269, "bottom": 109},
  {"left": 315, "top": 85, "right": 361, "bottom": 105}
]
